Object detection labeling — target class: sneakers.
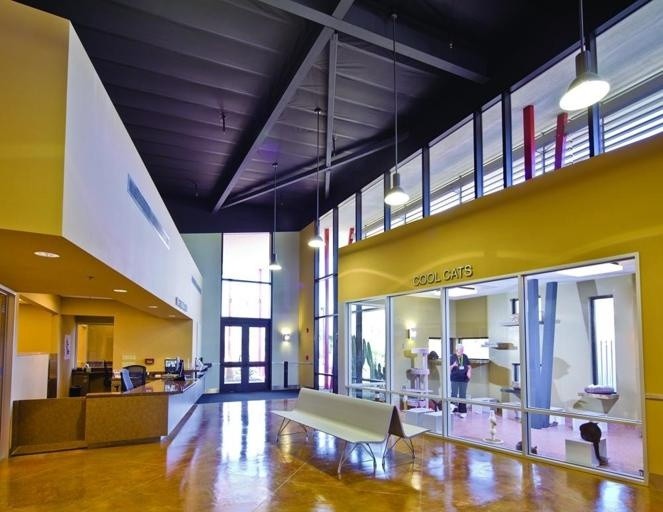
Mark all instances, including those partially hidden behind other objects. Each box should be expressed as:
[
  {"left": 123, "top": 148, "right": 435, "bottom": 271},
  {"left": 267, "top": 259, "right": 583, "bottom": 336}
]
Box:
[{"left": 450, "top": 403, "right": 468, "bottom": 418}]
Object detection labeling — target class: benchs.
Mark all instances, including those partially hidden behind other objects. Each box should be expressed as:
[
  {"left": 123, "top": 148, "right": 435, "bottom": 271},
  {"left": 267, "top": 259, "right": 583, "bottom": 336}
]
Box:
[{"left": 268, "top": 387, "right": 430, "bottom": 475}]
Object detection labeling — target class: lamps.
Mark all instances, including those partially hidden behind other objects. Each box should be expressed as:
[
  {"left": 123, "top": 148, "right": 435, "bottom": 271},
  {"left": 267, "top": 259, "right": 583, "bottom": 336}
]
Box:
[
  {"left": 306, "top": 106, "right": 328, "bottom": 251},
  {"left": 383, "top": 6, "right": 410, "bottom": 208},
  {"left": 558, "top": 1, "right": 611, "bottom": 115},
  {"left": 268, "top": 157, "right": 284, "bottom": 271}
]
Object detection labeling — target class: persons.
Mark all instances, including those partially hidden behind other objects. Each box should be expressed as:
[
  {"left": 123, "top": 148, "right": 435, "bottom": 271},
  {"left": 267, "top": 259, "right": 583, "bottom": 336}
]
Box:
[{"left": 450, "top": 343, "right": 472, "bottom": 418}]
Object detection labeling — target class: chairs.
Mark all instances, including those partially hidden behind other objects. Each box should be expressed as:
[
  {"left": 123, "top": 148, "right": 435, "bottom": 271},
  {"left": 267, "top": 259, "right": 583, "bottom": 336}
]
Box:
[{"left": 120, "top": 364, "right": 146, "bottom": 392}]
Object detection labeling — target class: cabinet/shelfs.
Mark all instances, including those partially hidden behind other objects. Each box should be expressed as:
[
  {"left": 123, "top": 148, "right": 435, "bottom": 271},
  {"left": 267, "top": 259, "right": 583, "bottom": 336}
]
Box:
[{"left": 72, "top": 369, "right": 111, "bottom": 393}]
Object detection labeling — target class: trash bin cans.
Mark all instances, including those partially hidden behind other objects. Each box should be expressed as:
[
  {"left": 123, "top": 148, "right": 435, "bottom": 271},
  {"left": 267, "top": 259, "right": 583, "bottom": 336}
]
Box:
[{"left": 70, "top": 385, "right": 81, "bottom": 397}]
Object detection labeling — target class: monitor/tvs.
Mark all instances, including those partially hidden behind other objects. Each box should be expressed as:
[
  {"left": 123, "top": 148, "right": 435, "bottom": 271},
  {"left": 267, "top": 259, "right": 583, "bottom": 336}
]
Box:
[
  {"left": 458, "top": 337, "right": 489, "bottom": 360},
  {"left": 427, "top": 336, "right": 453, "bottom": 360},
  {"left": 174, "top": 355, "right": 181, "bottom": 372}
]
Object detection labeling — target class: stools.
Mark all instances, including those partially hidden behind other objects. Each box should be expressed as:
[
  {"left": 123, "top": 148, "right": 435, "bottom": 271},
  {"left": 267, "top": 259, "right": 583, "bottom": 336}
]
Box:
[{"left": 352, "top": 382, "right": 609, "bottom": 469}]
